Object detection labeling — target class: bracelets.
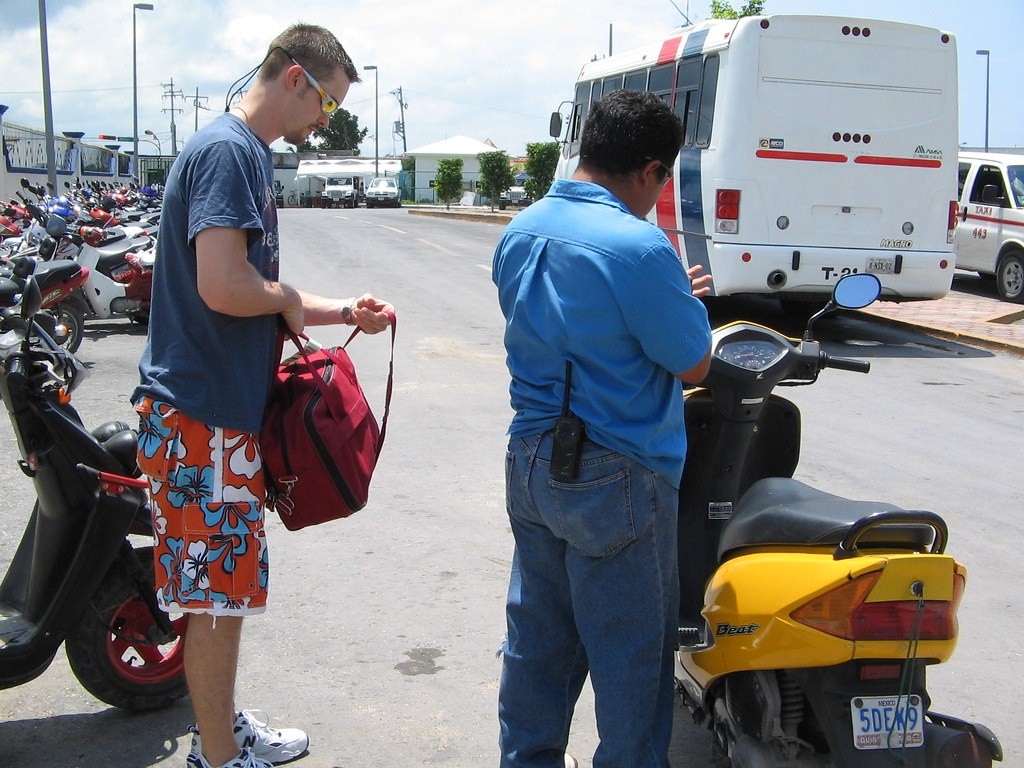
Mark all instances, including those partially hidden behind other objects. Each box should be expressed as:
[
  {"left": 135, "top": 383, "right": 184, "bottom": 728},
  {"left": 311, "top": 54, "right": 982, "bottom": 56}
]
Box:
[{"left": 342, "top": 297, "right": 353, "bottom": 327}]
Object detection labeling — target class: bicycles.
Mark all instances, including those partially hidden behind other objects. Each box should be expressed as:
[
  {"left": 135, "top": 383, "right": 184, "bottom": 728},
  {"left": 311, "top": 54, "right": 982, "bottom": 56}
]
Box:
[{"left": 288, "top": 189, "right": 306, "bottom": 206}]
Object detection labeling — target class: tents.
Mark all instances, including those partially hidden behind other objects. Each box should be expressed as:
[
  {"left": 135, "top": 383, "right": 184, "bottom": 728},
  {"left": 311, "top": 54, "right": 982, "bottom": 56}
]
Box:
[{"left": 294, "top": 158, "right": 403, "bottom": 206}]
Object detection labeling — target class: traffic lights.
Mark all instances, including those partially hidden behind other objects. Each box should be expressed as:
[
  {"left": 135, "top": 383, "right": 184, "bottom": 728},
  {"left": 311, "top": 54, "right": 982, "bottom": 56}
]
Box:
[{"left": 99, "top": 135, "right": 116, "bottom": 140}]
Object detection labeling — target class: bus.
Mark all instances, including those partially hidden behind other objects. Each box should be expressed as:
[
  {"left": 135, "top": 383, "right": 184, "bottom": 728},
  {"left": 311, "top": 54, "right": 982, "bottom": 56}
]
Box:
[{"left": 545, "top": 13, "right": 960, "bottom": 317}]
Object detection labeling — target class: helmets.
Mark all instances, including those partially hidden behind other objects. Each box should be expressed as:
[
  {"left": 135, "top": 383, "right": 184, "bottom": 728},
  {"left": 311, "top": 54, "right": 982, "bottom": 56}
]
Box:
[{"left": 80, "top": 225, "right": 107, "bottom": 247}]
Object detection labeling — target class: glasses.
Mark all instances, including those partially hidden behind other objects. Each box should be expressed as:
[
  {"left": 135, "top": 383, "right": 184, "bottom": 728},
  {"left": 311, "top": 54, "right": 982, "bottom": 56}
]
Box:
[
  {"left": 290, "top": 57, "right": 338, "bottom": 116},
  {"left": 644, "top": 155, "right": 675, "bottom": 187}
]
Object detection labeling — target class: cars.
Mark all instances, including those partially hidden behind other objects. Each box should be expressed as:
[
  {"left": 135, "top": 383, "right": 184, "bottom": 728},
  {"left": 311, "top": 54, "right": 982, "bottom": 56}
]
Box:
[
  {"left": 955, "top": 152, "right": 1024, "bottom": 303},
  {"left": 366, "top": 176, "right": 402, "bottom": 207}
]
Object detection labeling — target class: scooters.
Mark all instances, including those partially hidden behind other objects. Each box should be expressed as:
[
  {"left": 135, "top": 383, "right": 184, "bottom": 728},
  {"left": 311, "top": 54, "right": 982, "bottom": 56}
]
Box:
[
  {"left": 0, "top": 274, "right": 187, "bottom": 713},
  {"left": 674, "top": 272, "right": 1005, "bottom": 767}
]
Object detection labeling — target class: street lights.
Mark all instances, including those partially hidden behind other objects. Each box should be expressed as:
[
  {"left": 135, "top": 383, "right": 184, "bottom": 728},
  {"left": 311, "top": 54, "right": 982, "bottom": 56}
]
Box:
[
  {"left": 145, "top": 130, "right": 161, "bottom": 156},
  {"left": 976, "top": 50, "right": 990, "bottom": 152},
  {"left": 363, "top": 65, "right": 379, "bottom": 176},
  {"left": 132, "top": 4, "right": 155, "bottom": 183}
]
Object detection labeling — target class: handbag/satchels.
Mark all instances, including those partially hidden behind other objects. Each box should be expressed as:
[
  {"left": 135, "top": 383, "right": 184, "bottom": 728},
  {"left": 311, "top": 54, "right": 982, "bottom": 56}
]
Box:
[{"left": 260, "top": 309, "right": 398, "bottom": 531}]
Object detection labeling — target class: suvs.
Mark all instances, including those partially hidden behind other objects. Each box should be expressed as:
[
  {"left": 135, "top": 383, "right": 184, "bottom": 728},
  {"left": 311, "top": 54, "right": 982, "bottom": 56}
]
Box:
[
  {"left": 274, "top": 180, "right": 284, "bottom": 208},
  {"left": 500, "top": 179, "right": 533, "bottom": 210},
  {"left": 321, "top": 175, "right": 360, "bottom": 209}
]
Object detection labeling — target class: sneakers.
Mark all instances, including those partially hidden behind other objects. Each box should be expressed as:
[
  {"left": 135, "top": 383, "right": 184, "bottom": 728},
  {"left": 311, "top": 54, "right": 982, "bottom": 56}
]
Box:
[
  {"left": 186, "top": 747, "right": 275, "bottom": 768},
  {"left": 188, "top": 709, "right": 309, "bottom": 763}
]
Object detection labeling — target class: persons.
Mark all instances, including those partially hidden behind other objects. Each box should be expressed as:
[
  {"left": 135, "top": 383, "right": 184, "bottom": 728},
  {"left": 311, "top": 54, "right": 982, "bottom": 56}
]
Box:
[
  {"left": 488, "top": 90, "right": 714, "bottom": 768},
  {"left": 130, "top": 20, "right": 396, "bottom": 768}
]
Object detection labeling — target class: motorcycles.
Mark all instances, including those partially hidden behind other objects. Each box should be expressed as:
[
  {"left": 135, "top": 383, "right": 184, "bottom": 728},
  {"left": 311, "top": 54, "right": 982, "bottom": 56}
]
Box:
[{"left": 0, "top": 177, "right": 165, "bottom": 354}]
